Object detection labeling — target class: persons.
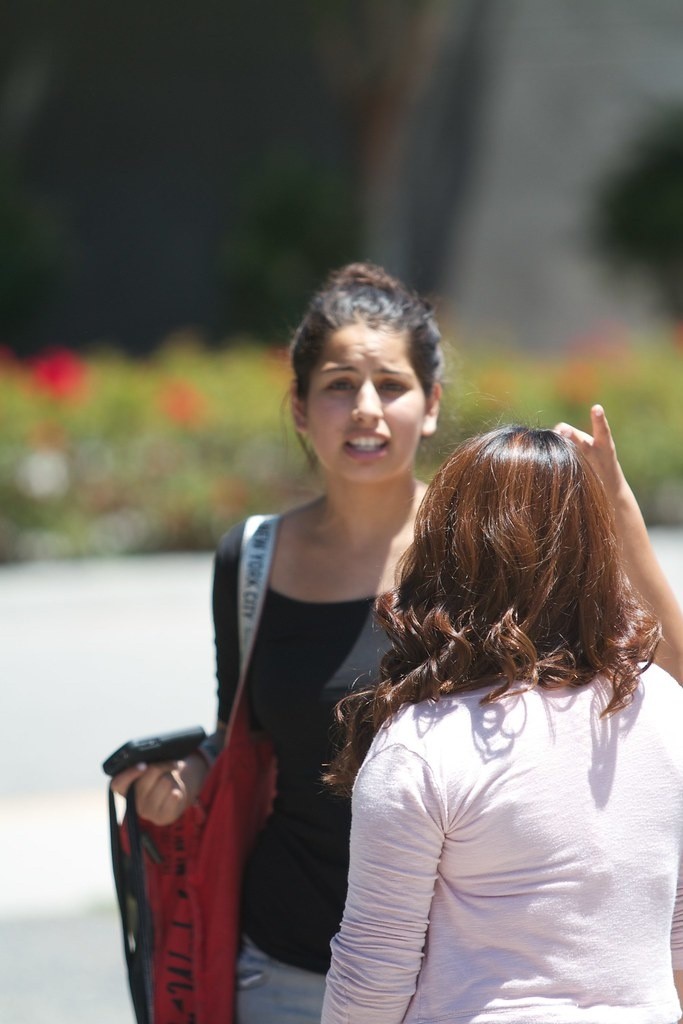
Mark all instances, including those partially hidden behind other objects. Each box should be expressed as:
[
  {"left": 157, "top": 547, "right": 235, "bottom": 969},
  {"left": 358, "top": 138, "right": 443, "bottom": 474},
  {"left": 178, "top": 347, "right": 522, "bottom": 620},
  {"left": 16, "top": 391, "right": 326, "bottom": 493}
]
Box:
[
  {"left": 319, "top": 424, "right": 683, "bottom": 1024},
  {"left": 105, "top": 261, "right": 683, "bottom": 1024}
]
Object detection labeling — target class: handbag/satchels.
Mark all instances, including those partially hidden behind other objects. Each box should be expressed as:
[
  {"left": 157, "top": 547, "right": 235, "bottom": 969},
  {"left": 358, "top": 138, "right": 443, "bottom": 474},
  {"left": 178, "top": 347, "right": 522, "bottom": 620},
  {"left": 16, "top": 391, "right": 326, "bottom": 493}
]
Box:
[{"left": 121, "top": 515, "right": 279, "bottom": 1024}]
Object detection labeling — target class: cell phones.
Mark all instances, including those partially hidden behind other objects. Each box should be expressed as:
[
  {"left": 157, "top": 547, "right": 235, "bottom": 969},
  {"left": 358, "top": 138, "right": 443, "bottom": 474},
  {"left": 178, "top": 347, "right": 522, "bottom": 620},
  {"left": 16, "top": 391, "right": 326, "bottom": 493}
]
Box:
[{"left": 103, "top": 726, "right": 207, "bottom": 776}]
